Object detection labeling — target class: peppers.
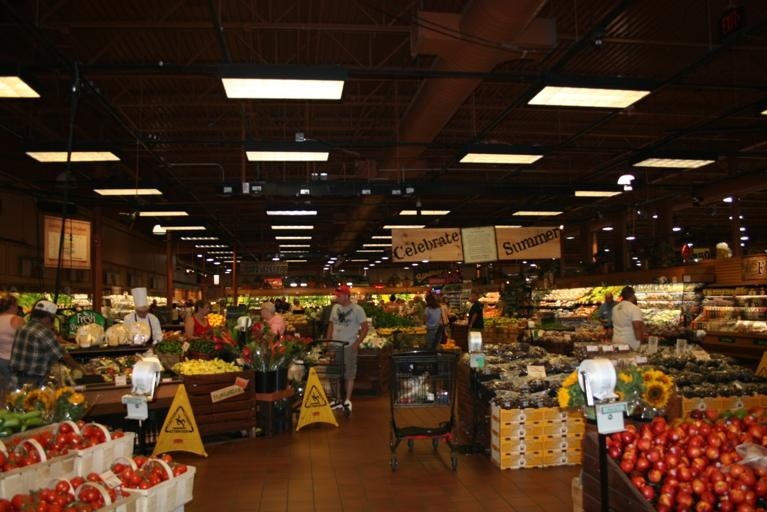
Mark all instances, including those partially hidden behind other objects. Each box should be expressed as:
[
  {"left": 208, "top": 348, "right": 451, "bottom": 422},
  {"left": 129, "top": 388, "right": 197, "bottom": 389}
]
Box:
[{"left": 0, "top": 409, "right": 43, "bottom": 437}]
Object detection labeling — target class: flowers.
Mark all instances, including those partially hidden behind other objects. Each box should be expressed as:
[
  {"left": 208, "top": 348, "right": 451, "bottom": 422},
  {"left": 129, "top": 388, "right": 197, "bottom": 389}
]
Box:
[
  {"left": 209, "top": 316, "right": 312, "bottom": 372},
  {"left": 556, "top": 360, "right": 676, "bottom": 416},
  {"left": 6, "top": 380, "right": 87, "bottom": 424}
]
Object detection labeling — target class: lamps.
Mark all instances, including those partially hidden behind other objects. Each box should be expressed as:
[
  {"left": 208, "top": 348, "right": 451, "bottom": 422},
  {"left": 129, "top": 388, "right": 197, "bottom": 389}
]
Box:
[
  {"left": 217, "top": 48, "right": 347, "bottom": 164},
  {"left": 631, "top": 147, "right": 718, "bottom": 170},
  {"left": 524, "top": 72, "right": 650, "bottom": 112},
  {"left": 456, "top": 92, "right": 546, "bottom": 166}
]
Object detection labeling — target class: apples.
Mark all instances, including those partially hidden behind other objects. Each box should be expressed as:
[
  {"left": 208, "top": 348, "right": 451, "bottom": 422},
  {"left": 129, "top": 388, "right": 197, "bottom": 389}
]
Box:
[{"left": 606, "top": 407, "right": 766, "bottom": 512}]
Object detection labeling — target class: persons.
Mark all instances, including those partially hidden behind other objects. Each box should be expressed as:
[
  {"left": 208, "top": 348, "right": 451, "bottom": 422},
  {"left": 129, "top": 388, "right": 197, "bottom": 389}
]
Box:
[
  {"left": 598, "top": 289, "right": 618, "bottom": 332},
  {"left": 323, "top": 284, "right": 369, "bottom": 413},
  {"left": 10, "top": 300, "right": 93, "bottom": 388},
  {"left": 611, "top": 285, "right": 649, "bottom": 347},
  {"left": 12, "top": 298, "right": 84, "bottom": 369},
  {"left": 0, "top": 295, "right": 27, "bottom": 387},
  {"left": 147, "top": 291, "right": 485, "bottom": 354},
  {"left": 461, "top": 226, "right": 497, "bottom": 264},
  {"left": 122, "top": 306, "right": 163, "bottom": 345}
]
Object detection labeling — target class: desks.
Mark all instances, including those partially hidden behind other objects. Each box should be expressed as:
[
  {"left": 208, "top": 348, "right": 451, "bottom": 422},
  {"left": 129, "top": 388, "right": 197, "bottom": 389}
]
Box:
[{"left": 256, "top": 388, "right": 295, "bottom": 437}]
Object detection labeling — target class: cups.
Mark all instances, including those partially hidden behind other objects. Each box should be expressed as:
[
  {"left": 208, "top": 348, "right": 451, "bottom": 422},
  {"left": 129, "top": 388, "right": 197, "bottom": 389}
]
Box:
[
  {"left": 676, "top": 339, "right": 686, "bottom": 358},
  {"left": 647, "top": 336, "right": 658, "bottom": 355}
]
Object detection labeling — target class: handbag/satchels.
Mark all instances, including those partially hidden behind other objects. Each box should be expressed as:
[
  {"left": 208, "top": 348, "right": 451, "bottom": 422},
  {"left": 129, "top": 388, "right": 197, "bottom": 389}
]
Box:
[{"left": 439, "top": 325, "right": 448, "bottom": 344}]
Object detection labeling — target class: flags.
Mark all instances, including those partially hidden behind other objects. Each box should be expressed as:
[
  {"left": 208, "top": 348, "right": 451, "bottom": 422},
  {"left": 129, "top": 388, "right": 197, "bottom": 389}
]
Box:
[
  {"left": 494, "top": 226, "right": 561, "bottom": 260},
  {"left": 391, "top": 227, "right": 464, "bottom": 263}
]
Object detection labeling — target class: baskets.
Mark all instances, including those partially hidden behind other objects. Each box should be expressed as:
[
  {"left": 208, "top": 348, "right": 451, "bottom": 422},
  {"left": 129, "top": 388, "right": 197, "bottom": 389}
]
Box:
[{"left": 1, "top": 420, "right": 196, "bottom": 511}]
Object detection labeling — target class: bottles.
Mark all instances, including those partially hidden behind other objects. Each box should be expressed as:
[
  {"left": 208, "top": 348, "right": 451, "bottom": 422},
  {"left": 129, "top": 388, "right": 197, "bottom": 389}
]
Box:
[{"left": 704, "top": 307, "right": 720, "bottom": 320}]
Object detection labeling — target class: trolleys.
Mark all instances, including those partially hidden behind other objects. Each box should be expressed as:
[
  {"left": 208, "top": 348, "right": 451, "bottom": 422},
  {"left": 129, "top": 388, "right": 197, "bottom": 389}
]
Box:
[
  {"left": 290, "top": 337, "right": 352, "bottom": 414},
  {"left": 380, "top": 350, "right": 463, "bottom": 473}
]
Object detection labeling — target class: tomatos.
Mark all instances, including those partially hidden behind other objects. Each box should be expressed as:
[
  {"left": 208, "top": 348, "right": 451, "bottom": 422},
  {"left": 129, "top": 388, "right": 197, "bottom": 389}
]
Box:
[
  {"left": 0, "top": 453, "right": 185, "bottom": 512},
  {"left": 0, "top": 420, "right": 127, "bottom": 477}
]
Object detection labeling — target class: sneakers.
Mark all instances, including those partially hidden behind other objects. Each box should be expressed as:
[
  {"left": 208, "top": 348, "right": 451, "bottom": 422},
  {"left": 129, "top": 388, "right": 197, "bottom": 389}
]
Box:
[
  {"left": 343, "top": 399, "right": 352, "bottom": 412},
  {"left": 329, "top": 401, "right": 344, "bottom": 410}
]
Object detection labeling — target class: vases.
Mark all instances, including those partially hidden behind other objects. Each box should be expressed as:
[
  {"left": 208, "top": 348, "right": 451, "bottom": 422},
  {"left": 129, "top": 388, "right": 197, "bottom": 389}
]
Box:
[
  {"left": 582, "top": 405, "right": 598, "bottom": 421},
  {"left": 255, "top": 367, "right": 288, "bottom": 393}
]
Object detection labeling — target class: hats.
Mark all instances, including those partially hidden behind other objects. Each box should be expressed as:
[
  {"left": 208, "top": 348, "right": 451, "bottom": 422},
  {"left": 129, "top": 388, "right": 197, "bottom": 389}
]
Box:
[
  {"left": 335, "top": 285, "right": 351, "bottom": 297},
  {"left": 34, "top": 300, "right": 59, "bottom": 315},
  {"left": 130, "top": 287, "right": 148, "bottom": 308}
]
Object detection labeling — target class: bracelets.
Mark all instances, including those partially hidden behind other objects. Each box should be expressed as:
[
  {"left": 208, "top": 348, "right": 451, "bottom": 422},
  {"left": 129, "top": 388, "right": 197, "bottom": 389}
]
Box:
[{"left": 326, "top": 334, "right": 331, "bottom": 339}]
